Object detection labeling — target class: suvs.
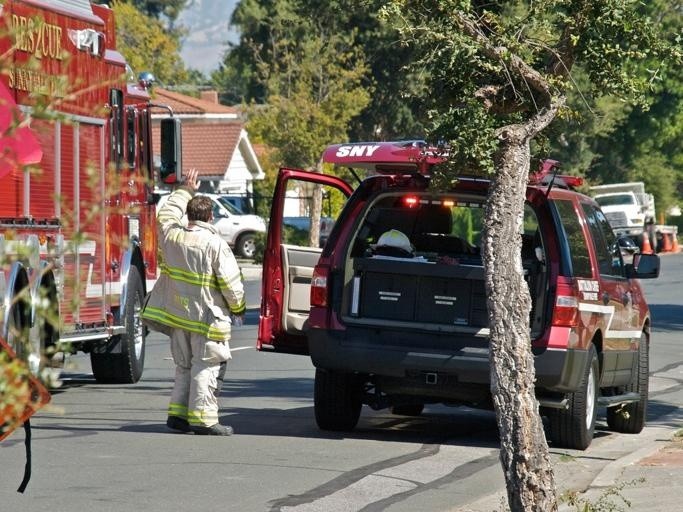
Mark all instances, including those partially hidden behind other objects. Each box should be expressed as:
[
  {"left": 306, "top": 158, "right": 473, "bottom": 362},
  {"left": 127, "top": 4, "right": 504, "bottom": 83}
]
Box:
[{"left": 255, "top": 140, "right": 661, "bottom": 451}]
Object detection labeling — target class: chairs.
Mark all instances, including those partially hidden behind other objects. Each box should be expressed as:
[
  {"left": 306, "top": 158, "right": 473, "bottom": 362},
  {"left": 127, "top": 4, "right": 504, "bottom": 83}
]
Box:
[{"left": 416, "top": 203, "right": 470, "bottom": 264}]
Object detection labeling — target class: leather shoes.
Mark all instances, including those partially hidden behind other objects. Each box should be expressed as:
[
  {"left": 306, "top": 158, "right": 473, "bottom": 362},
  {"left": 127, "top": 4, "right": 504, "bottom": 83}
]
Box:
[
  {"left": 167, "top": 416, "right": 194, "bottom": 432},
  {"left": 192, "top": 423, "right": 233, "bottom": 435}
]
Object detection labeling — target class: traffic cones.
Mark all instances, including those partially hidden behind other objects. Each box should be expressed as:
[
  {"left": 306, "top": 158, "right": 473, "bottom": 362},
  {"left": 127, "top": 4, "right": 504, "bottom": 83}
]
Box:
[{"left": 641, "top": 225, "right": 680, "bottom": 254}]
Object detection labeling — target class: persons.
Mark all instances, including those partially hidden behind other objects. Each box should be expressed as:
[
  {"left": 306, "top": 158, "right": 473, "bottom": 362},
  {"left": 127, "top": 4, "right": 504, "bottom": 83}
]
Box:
[{"left": 139, "top": 166, "right": 247, "bottom": 437}]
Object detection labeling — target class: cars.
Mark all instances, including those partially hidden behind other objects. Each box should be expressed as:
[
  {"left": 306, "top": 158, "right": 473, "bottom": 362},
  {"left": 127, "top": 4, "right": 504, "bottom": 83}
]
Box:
[{"left": 281, "top": 216, "right": 336, "bottom": 250}]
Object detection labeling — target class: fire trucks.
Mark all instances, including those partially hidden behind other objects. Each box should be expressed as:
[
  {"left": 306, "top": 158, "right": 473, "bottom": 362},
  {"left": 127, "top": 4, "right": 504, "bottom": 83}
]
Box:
[{"left": 0, "top": 0, "right": 184, "bottom": 404}]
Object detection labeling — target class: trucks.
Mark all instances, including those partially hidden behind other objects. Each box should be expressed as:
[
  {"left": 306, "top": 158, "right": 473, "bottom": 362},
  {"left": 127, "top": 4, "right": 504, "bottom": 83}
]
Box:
[{"left": 588, "top": 181, "right": 658, "bottom": 256}]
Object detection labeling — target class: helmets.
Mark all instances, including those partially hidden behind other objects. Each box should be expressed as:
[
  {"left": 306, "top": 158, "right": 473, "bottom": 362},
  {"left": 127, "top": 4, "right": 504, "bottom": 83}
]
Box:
[{"left": 370, "top": 229, "right": 415, "bottom": 256}]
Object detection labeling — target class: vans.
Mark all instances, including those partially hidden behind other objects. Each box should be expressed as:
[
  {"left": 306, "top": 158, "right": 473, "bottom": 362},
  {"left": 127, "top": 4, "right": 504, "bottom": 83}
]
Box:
[{"left": 153, "top": 188, "right": 269, "bottom": 259}]
